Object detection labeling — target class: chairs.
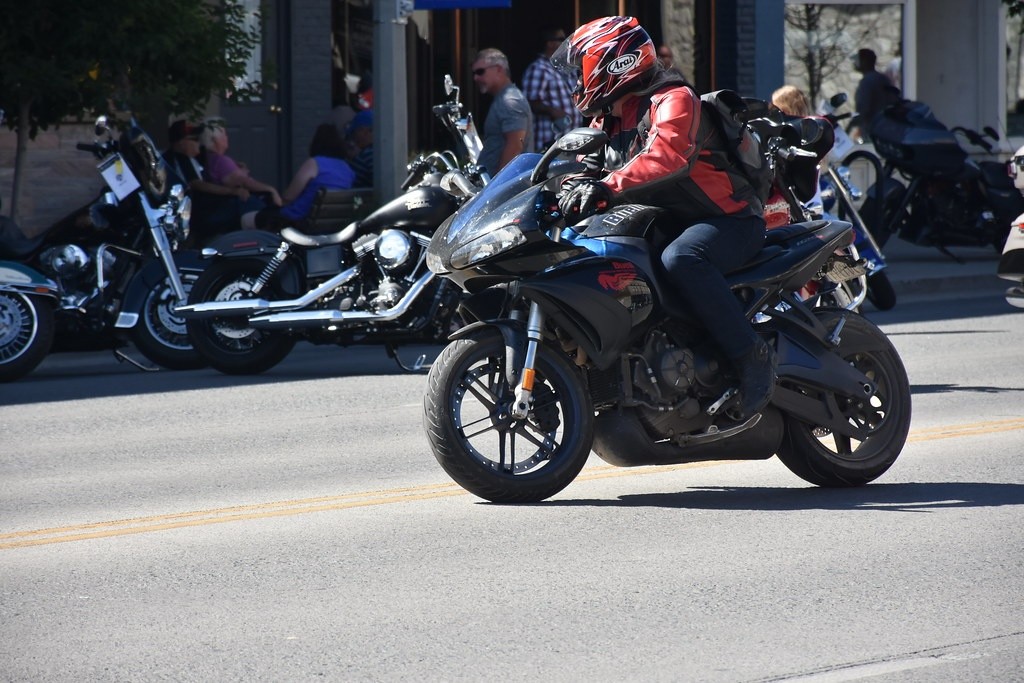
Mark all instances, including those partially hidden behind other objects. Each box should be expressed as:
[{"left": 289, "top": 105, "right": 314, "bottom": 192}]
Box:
[{"left": 301, "top": 187, "right": 374, "bottom": 236}]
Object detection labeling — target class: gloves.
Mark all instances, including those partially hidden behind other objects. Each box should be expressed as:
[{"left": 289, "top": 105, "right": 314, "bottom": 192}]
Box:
[{"left": 557, "top": 180, "right": 614, "bottom": 220}]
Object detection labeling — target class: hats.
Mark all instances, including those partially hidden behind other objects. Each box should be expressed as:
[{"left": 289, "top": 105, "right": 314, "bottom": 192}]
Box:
[
  {"left": 850, "top": 49, "right": 876, "bottom": 63},
  {"left": 168, "top": 120, "right": 203, "bottom": 141},
  {"left": 348, "top": 109, "right": 372, "bottom": 129}
]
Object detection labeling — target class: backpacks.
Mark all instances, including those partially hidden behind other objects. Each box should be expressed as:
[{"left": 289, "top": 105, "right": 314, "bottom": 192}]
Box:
[{"left": 700, "top": 88, "right": 836, "bottom": 208}]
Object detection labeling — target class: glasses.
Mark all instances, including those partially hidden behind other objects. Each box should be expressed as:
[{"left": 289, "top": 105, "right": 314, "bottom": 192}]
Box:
[
  {"left": 471, "top": 65, "right": 499, "bottom": 77},
  {"left": 184, "top": 136, "right": 200, "bottom": 143}
]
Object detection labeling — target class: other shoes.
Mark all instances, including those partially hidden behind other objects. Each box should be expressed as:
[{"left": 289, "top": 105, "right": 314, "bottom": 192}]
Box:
[{"left": 736, "top": 334, "right": 777, "bottom": 408}]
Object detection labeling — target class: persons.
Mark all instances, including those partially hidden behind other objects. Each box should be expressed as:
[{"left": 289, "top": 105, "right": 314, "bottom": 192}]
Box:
[
  {"left": 522, "top": 21, "right": 584, "bottom": 151},
  {"left": 769, "top": 84, "right": 810, "bottom": 117},
  {"left": 885, "top": 42, "right": 903, "bottom": 90},
  {"left": 344, "top": 109, "right": 375, "bottom": 188},
  {"left": 853, "top": 49, "right": 889, "bottom": 115},
  {"left": 470, "top": 47, "right": 535, "bottom": 179},
  {"left": 156, "top": 120, "right": 242, "bottom": 247},
  {"left": 548, "top": 16, "right": 777, "bottom": 428},
  {"left": 657, "top": 45, "right": 673, "bottom": 68},
  {"left": 240, "top": 124, "right": 355, "bottom": 231},
  {"left": 199, "top": 116, "right": 282, "bottom": 212}
]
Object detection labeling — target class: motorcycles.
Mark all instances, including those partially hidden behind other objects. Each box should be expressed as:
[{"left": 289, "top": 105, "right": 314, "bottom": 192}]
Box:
[
  {"left": 424, "top": 127, "right": 911, "bottom": 501},
  {"left": 175, "top": 75, "right": 484, "bottom": 377},
  {"left": 732, "top": 92, "right": 1024, "bottom": 310},
  {"left": 0, "top": 96, "right": 214, "bottom": 383}
]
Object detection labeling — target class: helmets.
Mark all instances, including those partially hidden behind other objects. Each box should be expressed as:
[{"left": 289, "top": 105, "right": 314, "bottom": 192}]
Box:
[{"left": 548, "top": 15, "right": 658, "bottom": 117}]
